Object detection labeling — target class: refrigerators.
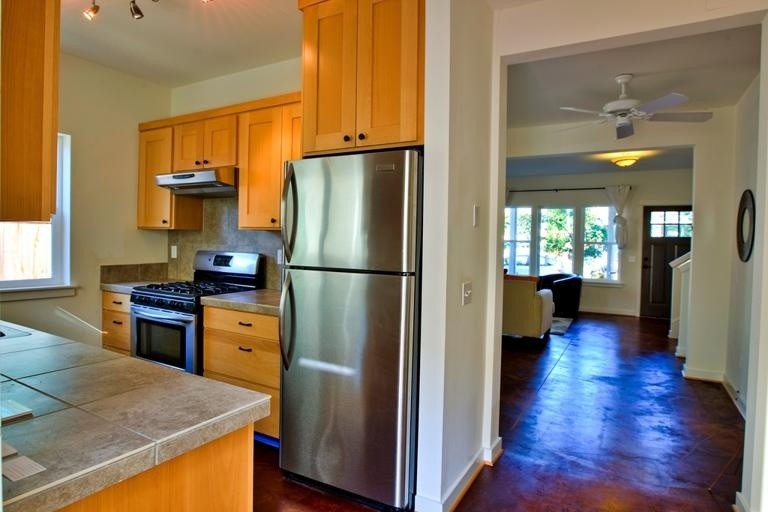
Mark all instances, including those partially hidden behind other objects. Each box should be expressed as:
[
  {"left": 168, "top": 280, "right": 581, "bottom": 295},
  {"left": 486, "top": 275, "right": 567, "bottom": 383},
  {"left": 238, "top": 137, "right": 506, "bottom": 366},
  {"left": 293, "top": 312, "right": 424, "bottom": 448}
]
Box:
[{"left": 273, "top": 147, "right": 424, "bottom": 511}]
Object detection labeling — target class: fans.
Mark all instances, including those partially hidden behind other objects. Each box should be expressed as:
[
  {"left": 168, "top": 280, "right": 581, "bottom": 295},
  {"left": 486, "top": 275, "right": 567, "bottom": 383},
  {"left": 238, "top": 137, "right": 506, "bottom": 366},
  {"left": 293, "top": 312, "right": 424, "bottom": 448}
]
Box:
[{"left": 548, "top": 73, "right": 714, "bottom": 141}]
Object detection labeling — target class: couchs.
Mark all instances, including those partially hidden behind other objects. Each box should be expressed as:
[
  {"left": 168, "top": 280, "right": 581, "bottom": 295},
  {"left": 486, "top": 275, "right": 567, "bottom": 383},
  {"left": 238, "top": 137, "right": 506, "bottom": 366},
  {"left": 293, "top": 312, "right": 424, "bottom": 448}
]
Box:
[
  {"left": 536, "top": 273, "right": 582, "bottom": 318},
  {"left": 502, "top": 273, "right": 554, "bottom": 341}
]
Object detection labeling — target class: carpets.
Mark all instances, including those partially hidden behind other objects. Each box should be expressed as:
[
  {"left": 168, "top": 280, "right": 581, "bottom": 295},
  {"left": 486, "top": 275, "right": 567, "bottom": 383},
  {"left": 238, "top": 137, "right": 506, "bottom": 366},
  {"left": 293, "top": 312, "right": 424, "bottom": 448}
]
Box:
[{"left": 549, "top": 315, "right": 575, "bottom": 336}]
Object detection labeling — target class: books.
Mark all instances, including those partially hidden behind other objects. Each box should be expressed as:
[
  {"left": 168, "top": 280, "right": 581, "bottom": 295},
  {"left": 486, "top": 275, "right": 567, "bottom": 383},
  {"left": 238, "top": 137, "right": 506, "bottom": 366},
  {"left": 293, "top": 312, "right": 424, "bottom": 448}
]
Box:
[{"left": 0, "top": 399, "right": 33, "bottom": 422}]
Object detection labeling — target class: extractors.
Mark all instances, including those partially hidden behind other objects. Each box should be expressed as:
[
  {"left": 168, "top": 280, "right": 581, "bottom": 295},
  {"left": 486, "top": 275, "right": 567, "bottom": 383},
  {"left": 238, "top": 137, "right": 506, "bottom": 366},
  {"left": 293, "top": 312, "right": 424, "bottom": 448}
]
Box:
[{"left": 152, "top": 165, "right": 237, "bottom": 200}]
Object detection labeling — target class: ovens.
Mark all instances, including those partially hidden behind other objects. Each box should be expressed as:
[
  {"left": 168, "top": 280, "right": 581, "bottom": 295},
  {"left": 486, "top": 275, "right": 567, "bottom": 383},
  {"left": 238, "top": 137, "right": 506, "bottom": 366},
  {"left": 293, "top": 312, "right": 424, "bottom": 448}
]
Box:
[{"left": 129, "top": 304, "right": 200, "bottom": 377}]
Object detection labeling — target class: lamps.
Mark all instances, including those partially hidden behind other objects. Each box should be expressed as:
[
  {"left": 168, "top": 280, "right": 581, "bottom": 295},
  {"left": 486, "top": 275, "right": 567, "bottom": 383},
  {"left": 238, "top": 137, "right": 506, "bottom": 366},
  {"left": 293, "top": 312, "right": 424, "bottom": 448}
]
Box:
[
  {"left": 79, "top": 0, "right": 218, "bottom": 23},
  {"left": 613, "top": 156, "right": 640, "bottom": 169}
]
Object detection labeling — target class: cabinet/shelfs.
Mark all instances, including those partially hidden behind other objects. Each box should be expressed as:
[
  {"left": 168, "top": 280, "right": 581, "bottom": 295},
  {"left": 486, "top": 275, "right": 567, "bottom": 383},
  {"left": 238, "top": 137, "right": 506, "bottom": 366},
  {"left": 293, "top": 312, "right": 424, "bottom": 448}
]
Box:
[
  {"left": 171, "top": 110, "right": 239, "bottom": 171},
  {"left": 136, "top": 127, "right": 205, "bottom": 232},
  {"left": 0, "top": 0, "right": 61, "bottom": 227},
  {"left": 298, "top": 0, "right": 424, "bottom": 158},
  {"left": 101, "top": 290, "right": 131, "bottom": 355},
  {"left": 241, "top": 100, "right": 301, "bottom": 230},
  {"left": 202, "top": 305, "right": 280, "bottom": 442}
]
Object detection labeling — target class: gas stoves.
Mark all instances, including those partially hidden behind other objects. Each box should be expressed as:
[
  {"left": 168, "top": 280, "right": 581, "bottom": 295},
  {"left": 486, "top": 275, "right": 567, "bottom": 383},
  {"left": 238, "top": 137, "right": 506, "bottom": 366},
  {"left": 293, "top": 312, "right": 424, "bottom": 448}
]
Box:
[{"left": 129, "top": 279, "right": 251, "bottom": 313}]
